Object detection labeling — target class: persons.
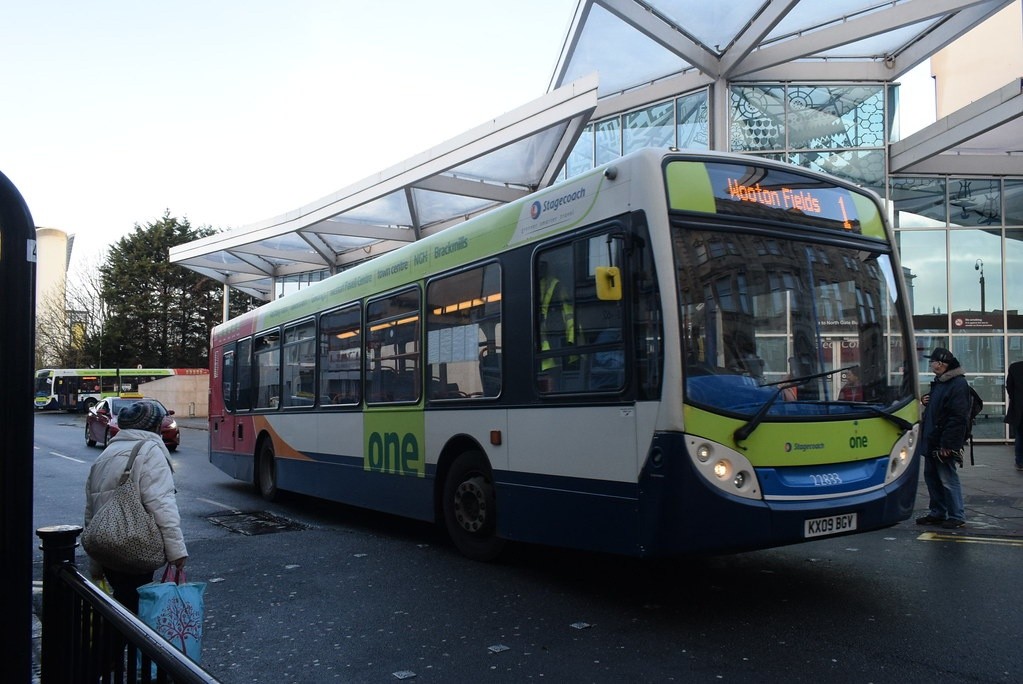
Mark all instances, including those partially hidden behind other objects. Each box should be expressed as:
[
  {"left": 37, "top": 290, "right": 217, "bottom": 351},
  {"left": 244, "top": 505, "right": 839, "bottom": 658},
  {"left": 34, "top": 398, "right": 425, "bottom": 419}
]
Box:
[
  {"left": 1006, "top": 361, "right": 1023, "bottom": 471},
  {"left": 85, "top": 400, "right": 189, "bottom": 684},
  {"left": 593, "top": 309, "right": 662, "bottom": 368},
  {"left": 838, "top": 366, "right": 863, "bottom": 402},
  {"left": 916, "top": 348, "right": 970, "bottom": 529},
  {"left": 727, "top": 330, "right": 763, "bottom": 375}
]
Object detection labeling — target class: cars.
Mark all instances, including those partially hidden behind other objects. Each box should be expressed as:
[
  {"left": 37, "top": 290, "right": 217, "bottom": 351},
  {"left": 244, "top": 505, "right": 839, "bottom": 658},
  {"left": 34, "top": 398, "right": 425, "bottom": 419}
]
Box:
[{"left": 84, "top": 392, "right": 180, "bottom": 453}]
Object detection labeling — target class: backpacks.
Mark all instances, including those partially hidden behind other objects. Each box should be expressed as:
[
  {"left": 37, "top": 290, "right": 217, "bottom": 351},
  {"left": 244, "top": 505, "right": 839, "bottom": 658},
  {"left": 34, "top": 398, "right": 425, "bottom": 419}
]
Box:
[{"left": 963, "top": 385, "right": 983, "bottom": 443}]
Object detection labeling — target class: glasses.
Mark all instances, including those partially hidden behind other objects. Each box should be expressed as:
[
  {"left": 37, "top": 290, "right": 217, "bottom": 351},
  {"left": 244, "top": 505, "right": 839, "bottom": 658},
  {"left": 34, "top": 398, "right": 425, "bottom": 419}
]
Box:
[{"left": 929, "top": 360, "right": 936, "bottom": 365}]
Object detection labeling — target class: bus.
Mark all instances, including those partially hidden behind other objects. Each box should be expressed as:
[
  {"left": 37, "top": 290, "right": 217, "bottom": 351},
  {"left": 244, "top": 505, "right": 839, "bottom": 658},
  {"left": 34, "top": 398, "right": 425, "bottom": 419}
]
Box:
[
  {"left": 207, "top": 146, "right": 932, "bottom": 566},
  {"left": 34, "top": 368, "right": 211, "bottom": 413}
]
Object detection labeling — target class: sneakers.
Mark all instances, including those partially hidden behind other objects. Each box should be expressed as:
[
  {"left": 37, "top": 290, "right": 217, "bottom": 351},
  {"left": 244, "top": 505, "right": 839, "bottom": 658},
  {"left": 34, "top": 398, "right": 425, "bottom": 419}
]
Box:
[
  {"left": 915, "top": 515, "right": 945, "bottom": 525},
  {"left": 942, "top": 518, "right": 964, "bottom": 528}
]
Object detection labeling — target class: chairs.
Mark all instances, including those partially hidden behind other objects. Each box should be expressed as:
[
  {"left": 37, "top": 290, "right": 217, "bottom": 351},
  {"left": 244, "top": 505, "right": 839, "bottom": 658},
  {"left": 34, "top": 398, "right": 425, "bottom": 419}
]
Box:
[{"left": 290, "top": 344, "right": 502, "bottom": 408}]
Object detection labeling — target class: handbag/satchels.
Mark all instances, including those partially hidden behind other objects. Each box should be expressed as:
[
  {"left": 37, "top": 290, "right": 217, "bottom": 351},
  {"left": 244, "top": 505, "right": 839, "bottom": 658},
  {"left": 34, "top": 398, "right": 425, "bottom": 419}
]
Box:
[
  {"left": 80, "top": 438, "right": 167, "bottom": 574},
  {"left": 136, "top": 562, "right": 207, "bottom": 680},
  {"left": 80, "top": 576, "right": 110, "bottom": 648}
]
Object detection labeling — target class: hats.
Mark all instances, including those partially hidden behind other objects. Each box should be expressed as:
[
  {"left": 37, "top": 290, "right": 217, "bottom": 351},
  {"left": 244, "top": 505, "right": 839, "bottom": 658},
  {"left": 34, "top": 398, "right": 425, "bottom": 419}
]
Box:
[
  {"left": 116, "top": 400, "right": 166, "bottom": 431},
  {"left": 923, "top": 347, "right": 953, "bottom": 364}
]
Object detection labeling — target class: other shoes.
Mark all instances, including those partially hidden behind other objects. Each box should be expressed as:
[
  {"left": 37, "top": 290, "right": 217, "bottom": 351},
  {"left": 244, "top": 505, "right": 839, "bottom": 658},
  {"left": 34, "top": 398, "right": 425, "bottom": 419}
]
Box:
[{"left": 1015, "top": 464, "right": 1023, "bottom": 470}]
